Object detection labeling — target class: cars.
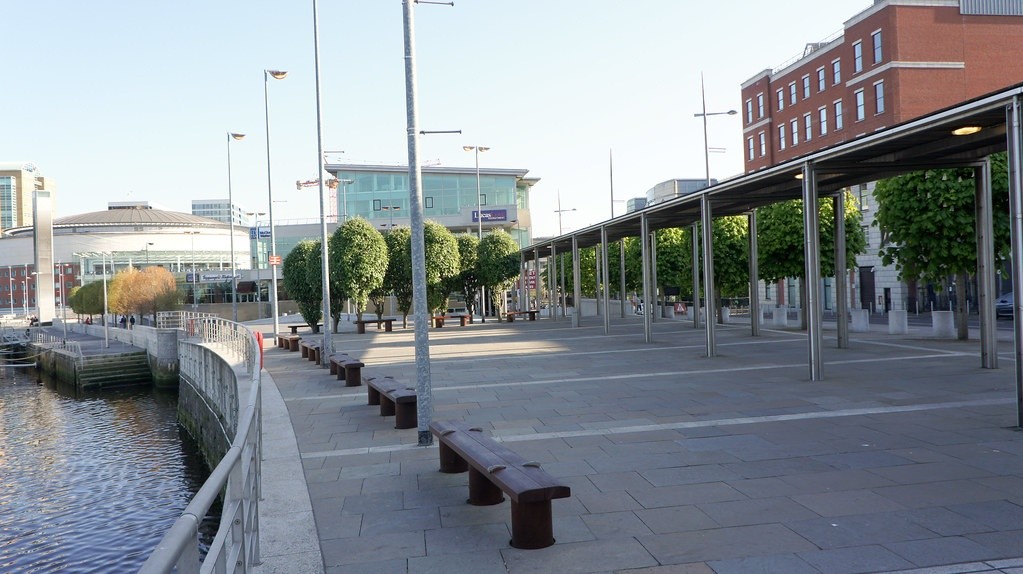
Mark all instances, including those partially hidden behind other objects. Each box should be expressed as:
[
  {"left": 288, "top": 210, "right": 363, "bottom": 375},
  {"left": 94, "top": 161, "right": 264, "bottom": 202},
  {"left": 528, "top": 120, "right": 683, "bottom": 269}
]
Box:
[{"left": 995, "top": 291, "right": 1013, "bottom": 320}]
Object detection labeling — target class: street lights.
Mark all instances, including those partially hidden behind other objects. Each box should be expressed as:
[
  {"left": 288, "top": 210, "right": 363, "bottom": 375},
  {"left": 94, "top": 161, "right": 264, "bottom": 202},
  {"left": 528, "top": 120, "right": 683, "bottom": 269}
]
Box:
[
  {"left": 399, "top": 0, "right": 455, "bottom": 446},
  {"left": 146, "top": 242, "right": 154, "bottom": 268},
  {"left": 227, "top": 131, "right": 247, "bottom": 338},
  {"left": 554, "top": 187, "right": 579, "bottom": 235},
  {"left": 185, "top": 231, "right": 200, "bottom": 317},
  {"left": 264, "top": 69, "right": 290, "bottom": 347},
  {"left": 694, "top": 69, "right": 738, "bottom": 188},
  {"left": 463, "top": 144, "right": 491, "bottom": 323}
]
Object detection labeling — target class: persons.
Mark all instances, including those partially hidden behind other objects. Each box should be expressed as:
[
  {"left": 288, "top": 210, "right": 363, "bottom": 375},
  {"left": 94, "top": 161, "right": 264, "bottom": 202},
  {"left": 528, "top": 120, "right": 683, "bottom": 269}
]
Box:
[
  {"left": 118, "top": 314, "right": 126, "bottom": 329},
  {"left": 130, "top": 316, "right": 135, "bottom": 326},
  {"left": 637, "top": 298, "right": 641, "bottom": 311},
  {"left": 86, "top": 317, "right": 90, "bottom": 325},
  {"left": 30, "top": 317, "right": 38, "bottom": 326},
  {"left": 26, "top": 329, "right": 30, "bottom": 337}
]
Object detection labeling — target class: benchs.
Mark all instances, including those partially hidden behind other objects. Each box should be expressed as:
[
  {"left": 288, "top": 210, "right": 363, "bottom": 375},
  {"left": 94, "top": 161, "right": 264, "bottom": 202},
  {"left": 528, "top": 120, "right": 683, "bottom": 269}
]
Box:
[
  {"left": 432, "top": 315, "right": 471, "bottom": 328},
  {"left": 361, "top": 373, "right": 417, "bottom": 429},
  {"left": 288, "top": 325, "right": 323, "bottom": 334},
  {"left": 502, "top": 310, "right": 539, "bottom": 323},
  {"left": 429, "top": 419, "right": 570, "bottom": 550},
  {"left": 328, "top": 351, "right": 364, "bottom": 387},
  {"left": 274, "top": 334, "right": 302, "bottom": 352},
  {"left": 353, "top": 319, "right": 397, "bottom": 334},
  {"left": 302, "top": 340, "right": 320, "bottom": 365}
]
